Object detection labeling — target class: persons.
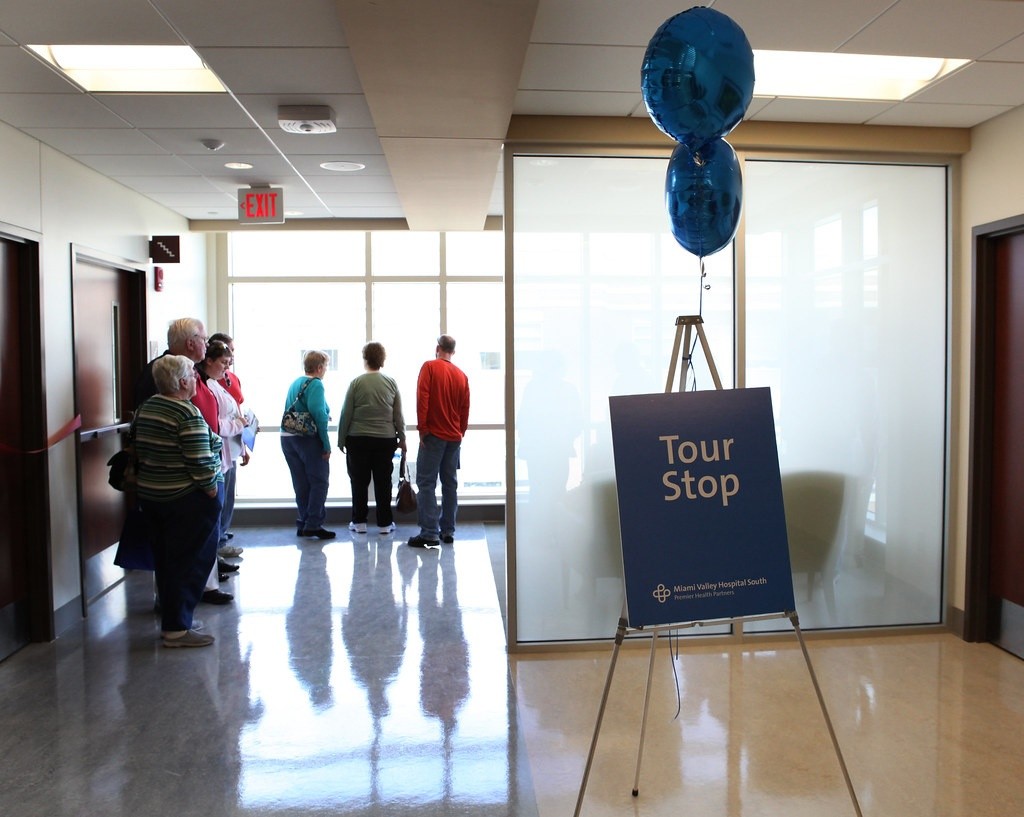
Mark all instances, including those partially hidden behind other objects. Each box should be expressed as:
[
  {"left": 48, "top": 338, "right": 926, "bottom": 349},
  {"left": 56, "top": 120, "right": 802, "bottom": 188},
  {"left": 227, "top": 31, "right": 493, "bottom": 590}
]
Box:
[
  {"left": 280, "top": 350, "right": 336, "bottom": 540},
  {"left": 338, "top": 342, "right": 407, "bottom": 534},
  {"left": 144, "top": 318, "right": 262, "bottom": 609},
  {"left": 408, "top": 335, "right": 470, "bottom": 547},
  {"left": 123, "top": 353, "right": 224, "bottom": 646}
]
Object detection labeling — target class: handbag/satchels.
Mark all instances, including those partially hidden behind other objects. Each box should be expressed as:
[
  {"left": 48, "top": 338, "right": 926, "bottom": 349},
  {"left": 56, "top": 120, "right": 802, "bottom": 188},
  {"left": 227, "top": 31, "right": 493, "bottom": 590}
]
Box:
[
  {"left": 281, "top": 377, "right": 321, "bottom": 438},
  {"left": 396, "top": 450, "right": 417, "bottom": 514},
  {"left": 108, "top": 404, "right": 144, "bottom": 493}
]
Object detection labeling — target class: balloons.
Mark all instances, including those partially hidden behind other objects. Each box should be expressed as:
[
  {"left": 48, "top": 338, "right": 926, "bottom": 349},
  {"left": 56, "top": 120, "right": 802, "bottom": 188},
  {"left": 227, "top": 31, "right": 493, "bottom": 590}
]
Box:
[
  {"left": 665, "top": 136, "right": 744, "bottom": 258},
  {"left": 641, "top": 7, "right": 756, "bottom": 154}
]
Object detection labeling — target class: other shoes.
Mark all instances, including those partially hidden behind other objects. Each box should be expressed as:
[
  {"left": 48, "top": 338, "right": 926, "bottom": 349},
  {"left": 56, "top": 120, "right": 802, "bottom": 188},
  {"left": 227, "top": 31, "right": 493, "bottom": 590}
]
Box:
[
  {"left": 163, "top": 629, "right": 215, "bottom": 647},
  {"left": 303, "top": 528, "right": 336, "bottom": 539},
  {"left": 408, "top": 536, "right": 439, "bottom": 549},
  {"left": 224, "top": 533, "right": 234, "bottom": 540},
  {"left": 438, "top": 531, "right": 453, "bottom": 543},
  {"left": 218, "top": 572, "right": 230, "bottom": 582},
  {"left": 199, "top": 589, "right": 234, "bottom": 605},
  {"left": 217, "top": 560, "right": 239, "bottom": 573},
  {"left": 160, "top": 620, "right": 204, "bottom": 637},
  {"left": 297, "top": 529, "right": 303, "bottom": 537},
  {"left": 218, "top": 545, "right": 243, "bottom": 558}
]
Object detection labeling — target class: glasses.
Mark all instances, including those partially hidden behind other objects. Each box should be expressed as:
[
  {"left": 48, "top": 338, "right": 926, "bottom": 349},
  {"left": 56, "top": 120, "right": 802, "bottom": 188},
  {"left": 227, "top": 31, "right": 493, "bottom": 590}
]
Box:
[
  {"left": 180, "top": 370, "right": 199, "bottom": 380},
  {"left": 194, "top": 333, "right": 210, "bottom": 344},
  {"left": 212, "top": 344, "right": 229, "bottom": 351}
]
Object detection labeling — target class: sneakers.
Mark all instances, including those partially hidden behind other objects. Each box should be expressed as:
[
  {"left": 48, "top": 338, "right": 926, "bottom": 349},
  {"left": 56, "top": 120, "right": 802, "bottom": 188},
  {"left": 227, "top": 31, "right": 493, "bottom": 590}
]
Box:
[
  {"left": 349, "top": 522, "right": 367, "bottom": 533},
  {"left": 378, "top": 522, "right": 396, "bottom": 534}
]
